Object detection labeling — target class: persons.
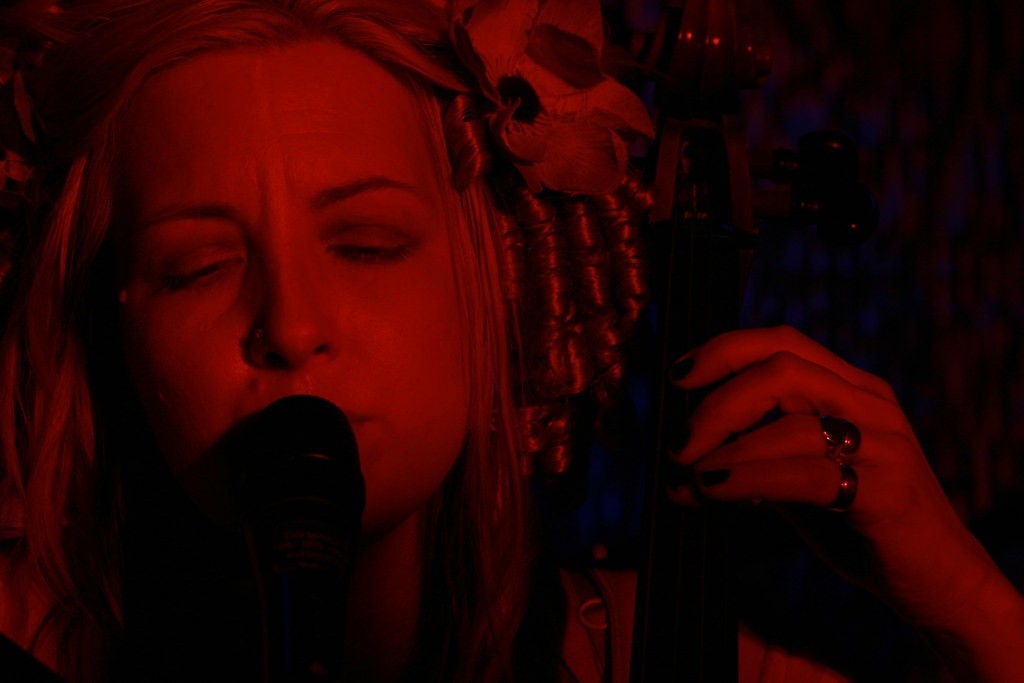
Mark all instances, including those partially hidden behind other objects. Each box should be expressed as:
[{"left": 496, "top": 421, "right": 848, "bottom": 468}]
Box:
[{"left": 2, "top": 0, "right": 1024, "bottom": 683}]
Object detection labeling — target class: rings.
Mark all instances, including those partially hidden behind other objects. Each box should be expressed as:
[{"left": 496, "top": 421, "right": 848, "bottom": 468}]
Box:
[
  {"left": 830, "top": 463, "right": 859, "bottom": 512},
  {"left": 821, "top": 413, "right": 861, "bottom": 462}
]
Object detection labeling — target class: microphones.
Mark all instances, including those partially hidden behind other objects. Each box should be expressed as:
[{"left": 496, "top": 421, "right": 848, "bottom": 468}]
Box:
[{"left": 197, "top": 392, "right": 365, "bottom": 623}]
